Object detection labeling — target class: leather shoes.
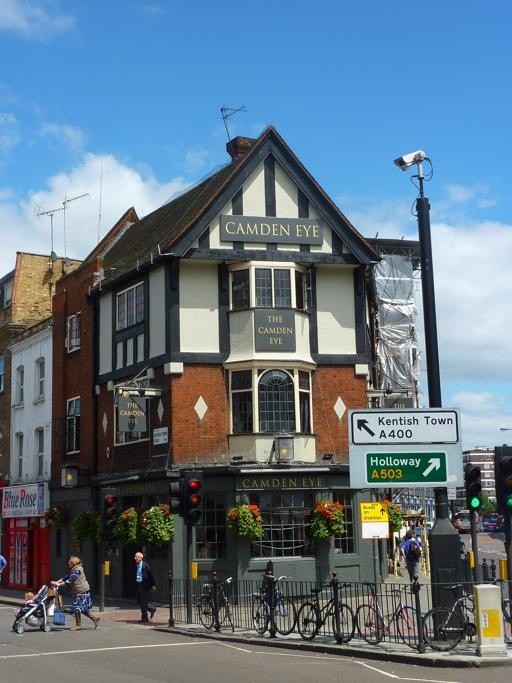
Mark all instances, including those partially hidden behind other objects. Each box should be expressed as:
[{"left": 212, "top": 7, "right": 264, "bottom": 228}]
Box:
[{"left": 138, "top": 608, "right": 156, "bottom": 623}]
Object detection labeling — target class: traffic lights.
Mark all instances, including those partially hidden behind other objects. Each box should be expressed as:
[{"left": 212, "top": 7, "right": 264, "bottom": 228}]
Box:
[
  {"left": 465, "top": 463, "right": 483, "bottom": 511},
  {"left": 98, "top": 486, "right": 120, "bottom": 537},
  {"left": 183, "top": 470, "right": 204, "bottom": 525},
  {"left": 495, "top": 444, "right": 512, "bottom": 514},
  {"left": 168, "top": 480, "right": 183, "bottom": 514}
]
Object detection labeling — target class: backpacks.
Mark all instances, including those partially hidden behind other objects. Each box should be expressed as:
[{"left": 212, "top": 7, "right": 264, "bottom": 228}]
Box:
[{"left": 408, "top": 540, "right": 421, "bottom": 559}]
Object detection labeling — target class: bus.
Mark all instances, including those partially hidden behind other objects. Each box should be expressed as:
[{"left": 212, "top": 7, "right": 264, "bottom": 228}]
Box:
[{"left": 458, "top": 509, "right": 480, "bottom": 533}]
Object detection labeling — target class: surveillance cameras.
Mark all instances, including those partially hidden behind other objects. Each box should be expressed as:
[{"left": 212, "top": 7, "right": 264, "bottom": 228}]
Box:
[{"left": 393, "top": 149, "right": 425, "bottom": 171}]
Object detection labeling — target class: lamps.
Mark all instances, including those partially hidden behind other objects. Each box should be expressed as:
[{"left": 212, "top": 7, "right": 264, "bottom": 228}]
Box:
[
  {"left": 107, "top": 380, "right": 114, "bottom": 391},
  {"left": 94, "top": 385, "right": 101, "bottom": 394},
  {"left": 147, "top": 368, "right": 154, "bottom": 380},
  {"left": 274, "top": 429, "right": 295, "bottom": 463},
  {"left": 62, "top": 464, "right": 79, "bottom": 489}
]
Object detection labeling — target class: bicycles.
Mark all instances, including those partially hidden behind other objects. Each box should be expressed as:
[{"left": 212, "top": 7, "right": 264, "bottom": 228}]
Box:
[
  {"left": 251, "top": 575, "right": 298, "bottom": 634},
  {"left": 356, "top": 585, "right": 429, "bottom": 650},
  {"left": 197, "top": 575, "right": 235, "bottom": 631},
  {"left": 296, "top": 583, "right": 356, "bottom": 643},
  {"left": 421, "top": 578, "right": 512, "bottom": 652}
]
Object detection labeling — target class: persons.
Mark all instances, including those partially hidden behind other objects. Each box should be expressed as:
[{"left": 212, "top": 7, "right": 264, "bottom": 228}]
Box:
[
  {"left": 0, "top": 554, "right": 8, "bottom": 581},
  {"left": 51, "top": 555, "right": 101, "bottom": 630},
  {"left": 134, "top": 551, "right": 157, "bottom": 622},
  {"left": 15, "top": 591, "right": 36, "bottom": 618},
  {"left": 399, "top": 530, "right": 416, "bottom": 569},
  {"left": 400, "top": 530, "right": 421, "bottom": 584}
]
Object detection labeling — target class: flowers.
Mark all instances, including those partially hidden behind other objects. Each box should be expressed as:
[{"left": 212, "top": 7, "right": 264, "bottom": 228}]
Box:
[
  {"left": 226, "top": 505, "right": 264, "bottom": 538},
  {"left": 45, "top": 506, "right": 176, "bottom": 544},
  {"left": 380, "top": 498, "right": 402, "bottom": 531},
  {"left": 310, "top": 500, "right": 346, "bottom": 542}
]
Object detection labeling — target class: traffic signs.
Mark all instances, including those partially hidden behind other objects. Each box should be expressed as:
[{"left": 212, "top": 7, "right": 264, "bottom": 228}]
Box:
[
  {"left": 349, "top": 407, "right": 463, "bottom": 487},
  {"left": 360, "top": 502, "right": 389, "bottom": 521}
]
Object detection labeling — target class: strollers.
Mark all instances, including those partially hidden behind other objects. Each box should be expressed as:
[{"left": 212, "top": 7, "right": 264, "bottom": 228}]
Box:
[{"left": 12, "top": 582, "right": 59, "bottom": 634}]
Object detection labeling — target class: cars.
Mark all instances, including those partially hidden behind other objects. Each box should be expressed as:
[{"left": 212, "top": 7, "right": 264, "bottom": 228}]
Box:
[{"left": 482, "top": 516, "right": 501, "bottom": 532}]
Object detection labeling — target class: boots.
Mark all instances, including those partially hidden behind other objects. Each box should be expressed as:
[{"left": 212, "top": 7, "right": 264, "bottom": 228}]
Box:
[
  {"left": 85, "top": 611, "right": 101, "bottom": 630},
  {"left": 70, "top": 612, "right": 82, "bottom": 631}
]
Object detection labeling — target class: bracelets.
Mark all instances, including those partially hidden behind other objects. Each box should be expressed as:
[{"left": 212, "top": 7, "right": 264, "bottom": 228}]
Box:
[{"left": 60, "top": 582, "right": 62, "bottom": 587}]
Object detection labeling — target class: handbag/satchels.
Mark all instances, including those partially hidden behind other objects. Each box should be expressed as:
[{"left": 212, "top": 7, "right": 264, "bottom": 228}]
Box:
[{"left": 53, "top": 609, "right": 66, "bottom": 625}]
